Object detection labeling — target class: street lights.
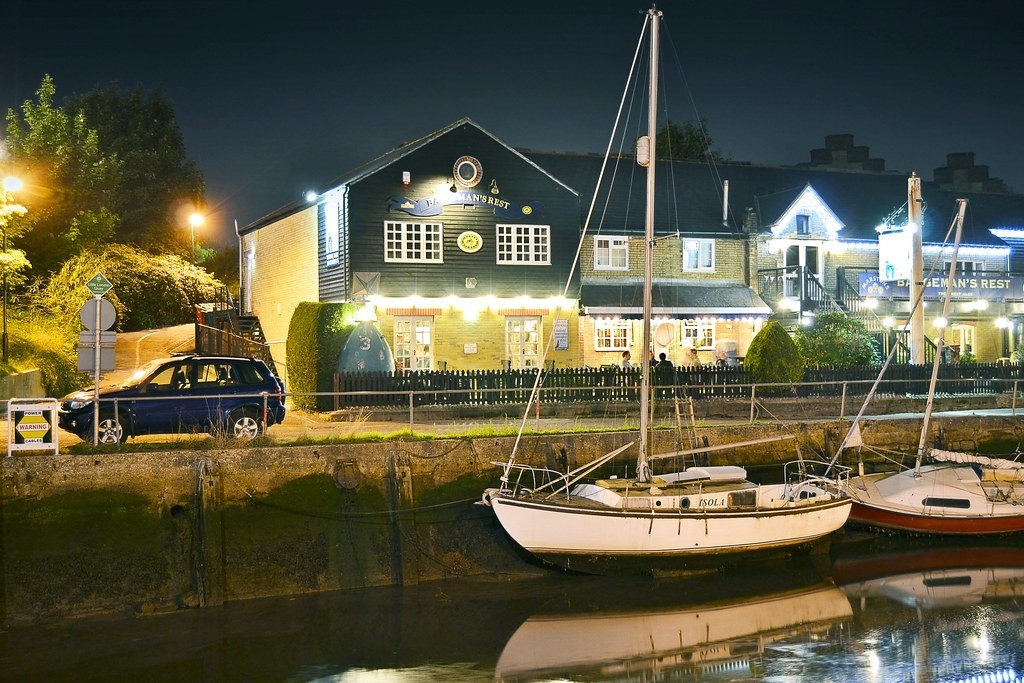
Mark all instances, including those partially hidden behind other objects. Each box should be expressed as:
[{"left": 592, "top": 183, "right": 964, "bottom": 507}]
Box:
[
  {"left": 189, "top": 214, "right": 201, "bottom": 268},
  {"left": 0, "top": 177, "right": 22, "bottom": 365}
]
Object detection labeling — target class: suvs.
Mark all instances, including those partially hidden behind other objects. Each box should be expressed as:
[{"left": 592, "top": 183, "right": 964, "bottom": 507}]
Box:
[{"left": 57, "top": 354, "right": 288, "bottom": 443}]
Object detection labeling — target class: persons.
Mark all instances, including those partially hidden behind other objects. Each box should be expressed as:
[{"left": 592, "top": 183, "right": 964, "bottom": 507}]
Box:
[
  {"left": 655, "top": 353, "right": 673, "bottom": 385},
  {"left": 618, "top": 350, "right": 634, "bottom": 372},
  {"left": 716, "top": 352, "right": 734, "bottom": 380},
  {"left": 687, "top": 348, "right": 702, "bottom": 383},
  {"left": 648, "top": 350, "right": 659, "bottom": 367}
]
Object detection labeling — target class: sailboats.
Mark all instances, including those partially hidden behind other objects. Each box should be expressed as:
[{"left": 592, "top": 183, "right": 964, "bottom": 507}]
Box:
[
  {"left": 493, "top": 565, "right": 854, "bottom": 683},
  {"left": 467, "top": 6, "right": 853, "bottom": 555},
  {"left": 825, "top": 190, "right": 1024, "bottom": 539}
]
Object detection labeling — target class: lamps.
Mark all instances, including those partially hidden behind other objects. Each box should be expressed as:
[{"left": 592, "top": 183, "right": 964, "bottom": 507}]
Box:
[
  {"left": 446, "top": 173, "right": 457, "bottom": 192},
  {"left": 490, "top": 179, "right": 499, "bottom": 194}
]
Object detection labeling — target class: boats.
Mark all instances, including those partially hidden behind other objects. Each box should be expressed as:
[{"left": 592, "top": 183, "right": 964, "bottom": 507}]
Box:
[{"left": 825, "top": 540, "right": 1024, "bottom": 611}]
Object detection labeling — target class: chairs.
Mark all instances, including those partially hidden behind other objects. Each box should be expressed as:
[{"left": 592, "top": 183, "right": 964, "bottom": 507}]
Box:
[
  {"left": 216, "top": 367, "right": 237, "bottom": 385},
  {"left": 174, "top": 370, "right": 194, "bottom": 389}
]
